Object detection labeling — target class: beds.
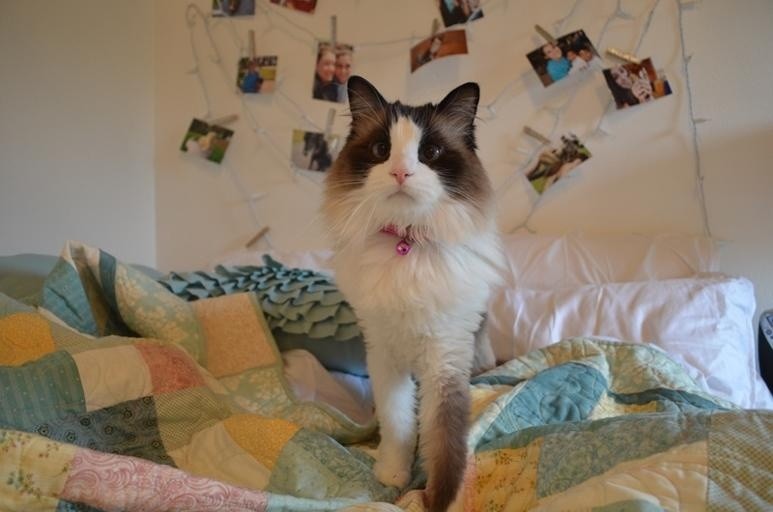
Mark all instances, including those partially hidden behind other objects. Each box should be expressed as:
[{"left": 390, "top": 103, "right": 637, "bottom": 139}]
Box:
[{"left": 1, "top": 247, "right": 773, "bottom": 512}]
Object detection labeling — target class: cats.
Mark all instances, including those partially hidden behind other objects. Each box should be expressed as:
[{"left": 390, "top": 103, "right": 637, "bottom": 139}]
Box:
[{"left": 317, "top": 73, "right": 509, "bottom": 512}]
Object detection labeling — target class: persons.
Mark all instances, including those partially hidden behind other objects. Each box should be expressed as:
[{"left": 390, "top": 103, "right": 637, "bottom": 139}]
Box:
[
  {"left": 418, "top": 35, "right": 442, "bottom": 67},
  {"left": 577, "top": 43, "right": 599, "bottom": 66},
  {"left": 561, "top": 46, "right": 586, "bottom": 74},
  {"left": 239, "top": 58, "right": 264, "bottom": 92},
  {"left": 183, "top": 126, "right": 220, "bottom": 159},
  {"left": 311, "top": 47, "right": 337, "bottom": 102},
  {"left": 601, "top": 65, "right": 654, "bottom": 110},
  {"left": 541, "top": 43, "right": 572, "bottom": 81},
  {"left": 323, "top": 49, "right": 351, "bottom": 103}
]
